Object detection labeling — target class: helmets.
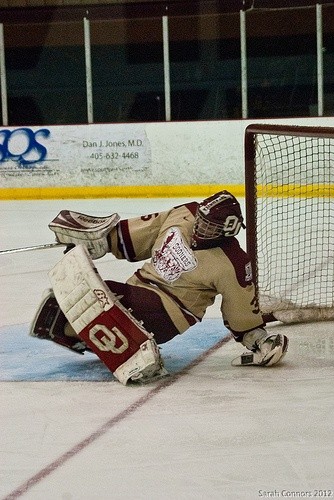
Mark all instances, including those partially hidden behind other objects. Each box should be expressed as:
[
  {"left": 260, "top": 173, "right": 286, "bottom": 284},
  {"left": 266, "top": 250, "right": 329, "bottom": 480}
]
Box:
[{"left": 191, "top": 190, "right": 243, "bottom": 242}]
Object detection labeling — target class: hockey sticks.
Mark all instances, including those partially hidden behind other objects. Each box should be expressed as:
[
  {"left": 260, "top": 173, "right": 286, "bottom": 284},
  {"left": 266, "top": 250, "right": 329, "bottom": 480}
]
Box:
[{"left": 0, "top": 242, "right": 66, "bottom": 255}]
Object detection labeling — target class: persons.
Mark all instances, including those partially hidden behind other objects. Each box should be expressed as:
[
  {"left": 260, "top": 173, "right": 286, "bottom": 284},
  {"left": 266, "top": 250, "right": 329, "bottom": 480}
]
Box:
[{"left": 28, "top": 191, "right": 289, "bottom": 384}]
